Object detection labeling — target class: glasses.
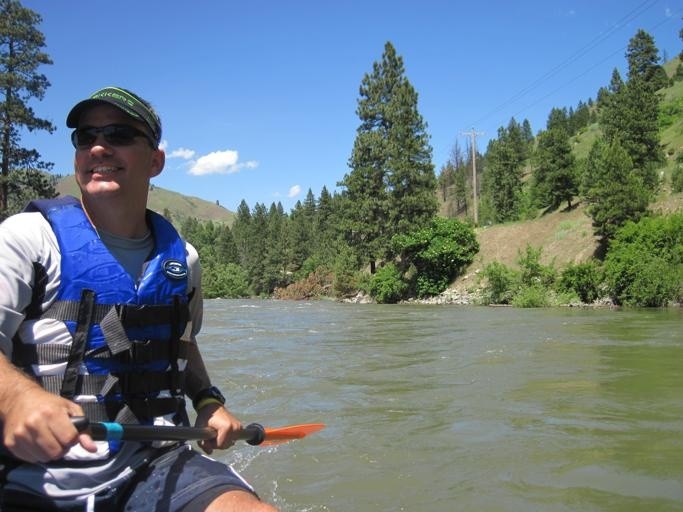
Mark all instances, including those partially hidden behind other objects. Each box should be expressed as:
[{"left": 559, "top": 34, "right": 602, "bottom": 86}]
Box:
[{"left": 71, "top": 123, "right": 157, "bottom": 151}]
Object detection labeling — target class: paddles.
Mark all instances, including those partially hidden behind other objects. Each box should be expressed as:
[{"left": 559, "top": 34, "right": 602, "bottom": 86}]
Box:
[{"left": 70, "top": 417, "right": 325, "bottom": 446}]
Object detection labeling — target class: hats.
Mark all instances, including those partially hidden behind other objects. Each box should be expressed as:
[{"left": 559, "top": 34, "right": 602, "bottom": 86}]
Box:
[{"left": 66, "top": 87, "right": 161, "bottom": 151}]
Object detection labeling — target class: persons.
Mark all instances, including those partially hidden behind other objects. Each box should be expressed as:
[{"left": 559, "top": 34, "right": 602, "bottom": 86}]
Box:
[{"left": 0, "top": 86, "right": 280, "bottom": 511}]
